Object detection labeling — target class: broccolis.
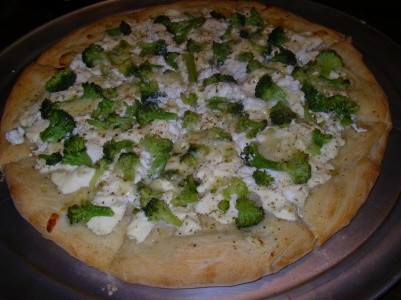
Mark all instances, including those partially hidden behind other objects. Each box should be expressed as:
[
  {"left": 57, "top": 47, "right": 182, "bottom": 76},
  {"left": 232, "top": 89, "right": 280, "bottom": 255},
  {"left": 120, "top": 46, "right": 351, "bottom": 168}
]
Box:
[{"left": 38, "top": 5, "right": 359, "bottom": 227}]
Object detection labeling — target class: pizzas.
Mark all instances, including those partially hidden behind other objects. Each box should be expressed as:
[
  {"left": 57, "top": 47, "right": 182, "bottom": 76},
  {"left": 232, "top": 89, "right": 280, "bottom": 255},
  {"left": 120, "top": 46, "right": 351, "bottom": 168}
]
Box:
[{"left": 1, "top": 0, "right": 394, "bottom": 289}]
